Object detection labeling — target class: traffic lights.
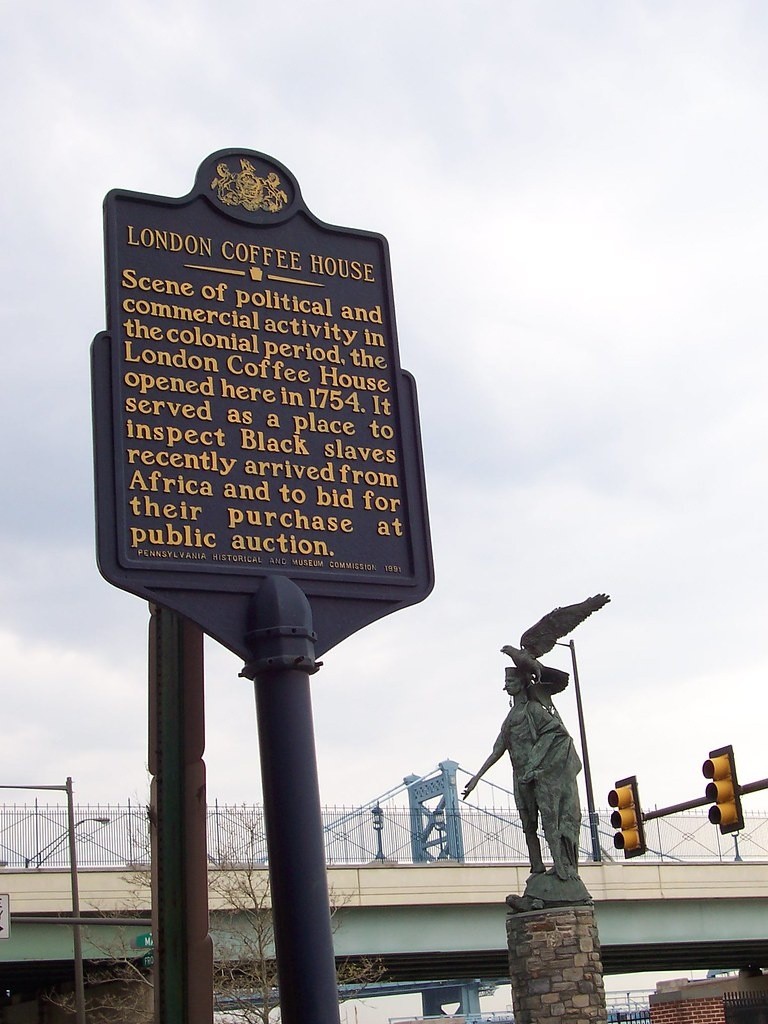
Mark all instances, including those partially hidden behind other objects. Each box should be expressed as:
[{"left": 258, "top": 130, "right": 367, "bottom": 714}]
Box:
[
  {"left": 607, "top": 775, "right": 647, "bottom": 860},
  {"left": 702, "top": 744, "right": 745, "bottom": 836}
]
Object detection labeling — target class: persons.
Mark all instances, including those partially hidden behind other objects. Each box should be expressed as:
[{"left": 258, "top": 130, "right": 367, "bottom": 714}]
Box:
[{"left": 461, "top": 667, "right": 570, "bottom": 882}]
